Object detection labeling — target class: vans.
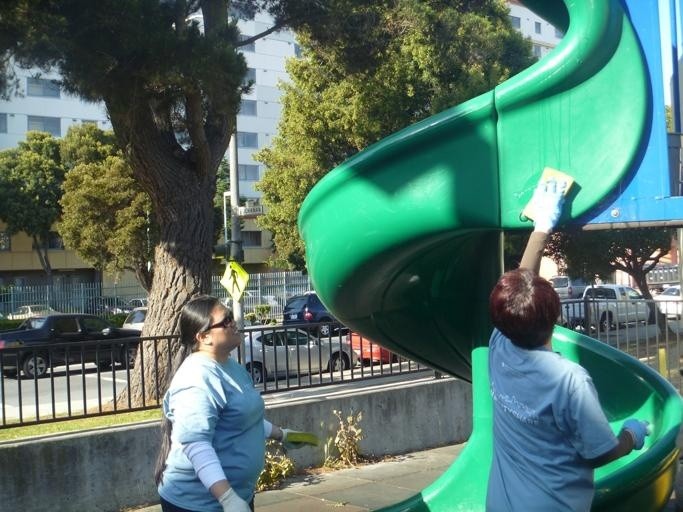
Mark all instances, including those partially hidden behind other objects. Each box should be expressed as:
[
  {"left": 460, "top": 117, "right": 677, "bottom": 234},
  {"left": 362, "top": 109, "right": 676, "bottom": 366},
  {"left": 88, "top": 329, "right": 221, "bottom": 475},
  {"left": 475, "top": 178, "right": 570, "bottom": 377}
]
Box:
[{"left": 548, "top": 275, "right": 598, "bottom": 301}]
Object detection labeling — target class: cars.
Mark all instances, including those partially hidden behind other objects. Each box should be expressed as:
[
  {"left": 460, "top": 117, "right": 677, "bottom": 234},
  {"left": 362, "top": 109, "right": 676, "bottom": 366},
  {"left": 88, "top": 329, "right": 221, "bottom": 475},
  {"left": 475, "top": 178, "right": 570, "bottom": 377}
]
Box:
[
  {"left": 347, "top": 331, "right": 410, "bottom": 367},
  {"left": 653, "top": 285, "right": 683, "bottom": 321},
  {"left": 6, "top": 296, "right": 150, "bottom": 331},
  {"left": 281, "top": 291, "right": 349, "bottom": 337},
  {"left": 229, "top": 326, "right": 360, "bottom": 386}
]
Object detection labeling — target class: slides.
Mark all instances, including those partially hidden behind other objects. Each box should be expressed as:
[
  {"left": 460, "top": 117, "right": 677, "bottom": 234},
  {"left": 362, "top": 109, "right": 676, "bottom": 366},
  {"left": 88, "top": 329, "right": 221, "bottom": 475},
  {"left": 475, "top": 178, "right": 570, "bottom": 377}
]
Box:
[{"left": 298, "top": 1, "right": 681, "bottom": 512}]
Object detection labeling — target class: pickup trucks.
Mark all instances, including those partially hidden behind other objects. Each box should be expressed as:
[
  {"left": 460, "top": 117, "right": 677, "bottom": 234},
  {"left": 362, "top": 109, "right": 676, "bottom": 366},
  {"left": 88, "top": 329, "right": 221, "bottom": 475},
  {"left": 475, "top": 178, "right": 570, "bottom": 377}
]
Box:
[
  {"left": 0, "top": 312, "right": 143, "bottom": 380},
  {"left": 557, "top": 284, "right": 655, "bottom": 335}
]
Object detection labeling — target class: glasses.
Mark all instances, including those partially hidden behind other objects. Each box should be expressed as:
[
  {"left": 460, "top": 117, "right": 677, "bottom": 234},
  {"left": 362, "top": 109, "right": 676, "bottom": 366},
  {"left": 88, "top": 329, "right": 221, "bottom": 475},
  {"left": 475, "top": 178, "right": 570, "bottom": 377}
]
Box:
[{"left": 206, "top": 311, "right": 234, "bottom": 332}]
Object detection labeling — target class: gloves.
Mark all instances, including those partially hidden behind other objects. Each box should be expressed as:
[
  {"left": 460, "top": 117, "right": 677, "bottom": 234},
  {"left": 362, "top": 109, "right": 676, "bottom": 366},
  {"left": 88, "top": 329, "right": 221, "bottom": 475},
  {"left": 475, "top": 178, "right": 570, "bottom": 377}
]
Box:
[
  {"left": 217, "top": 486, "right": 253, "bottom": 512},
  {"left": 620, "top": 416, "right": 651, "bottom": 452},
  {"left": 277, "top": 426, "right": 308, "bottom": 449},
  {"left": 533, "top": 178, "right": 568, "bottom": 236}
]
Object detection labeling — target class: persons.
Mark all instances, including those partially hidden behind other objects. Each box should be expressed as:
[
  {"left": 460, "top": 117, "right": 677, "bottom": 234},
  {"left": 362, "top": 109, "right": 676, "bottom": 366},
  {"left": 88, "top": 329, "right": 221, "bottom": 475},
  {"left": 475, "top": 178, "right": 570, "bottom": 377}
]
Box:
[
  {"left": 154, "top": 297, "right": 305, "bottom": 511},
  {"left": 483, "top": 179, "right": 654, "bottom": 511}
]
